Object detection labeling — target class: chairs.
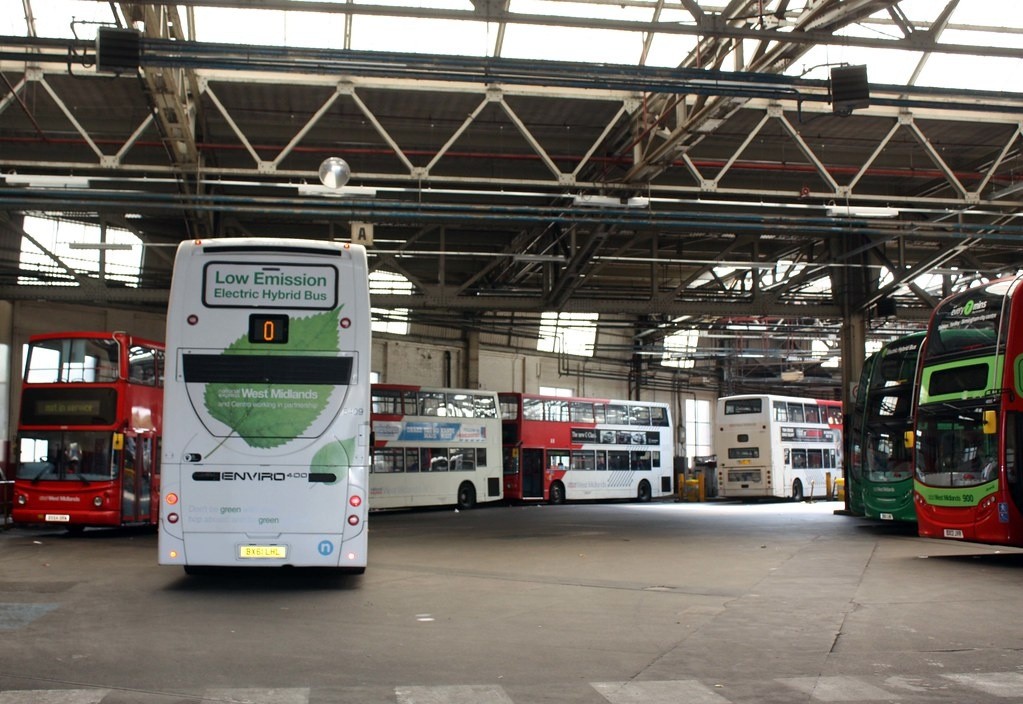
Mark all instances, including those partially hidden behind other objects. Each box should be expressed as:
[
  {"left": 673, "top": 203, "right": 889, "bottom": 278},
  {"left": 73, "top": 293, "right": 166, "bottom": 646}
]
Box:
[{"left": 53, "top": 450, "right": 109, "bottom": 476}]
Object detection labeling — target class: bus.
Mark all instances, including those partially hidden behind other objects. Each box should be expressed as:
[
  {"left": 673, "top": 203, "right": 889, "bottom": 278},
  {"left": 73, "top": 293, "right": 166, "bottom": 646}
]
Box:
[
  {"left": 845, "top": 273, "right": 1023, "bottom": 548},
  {"left": 369, "top": 384, "right": 504, "bottom": 513},
  {"left": 483, "top": 393, "right": 675, "bottom": 505},
  {"left": 10, "top": 330, "right": 165, "bottom": 536},
  {"left": 158, "top": 237, "right": 376, "bottom": 578},
  {"left": 712, "top": 394, "right": 889, "bottom": 502}
]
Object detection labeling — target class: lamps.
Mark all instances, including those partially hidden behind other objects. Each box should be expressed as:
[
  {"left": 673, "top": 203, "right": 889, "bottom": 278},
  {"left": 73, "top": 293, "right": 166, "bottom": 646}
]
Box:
[
  {"left": 25, "top": 66, "right": 43, "bottom": 81},
  {"left": 898, "top": 110, "right": 913, "bottom": 125},
  {"left": 766, "top": 102, "right": 783, "bottom": 118},
  {"left": 318, "top": 156, "right": 352, "bottom": 190},
  {"left": 831, "top": 63, "right": 869, "bottom": 115},
  {"left": 869, "top": 298, "right": 898, "bottom": 330},
  {"left": 93, "top": 26, "right": 143, "bottom": 74}
]
